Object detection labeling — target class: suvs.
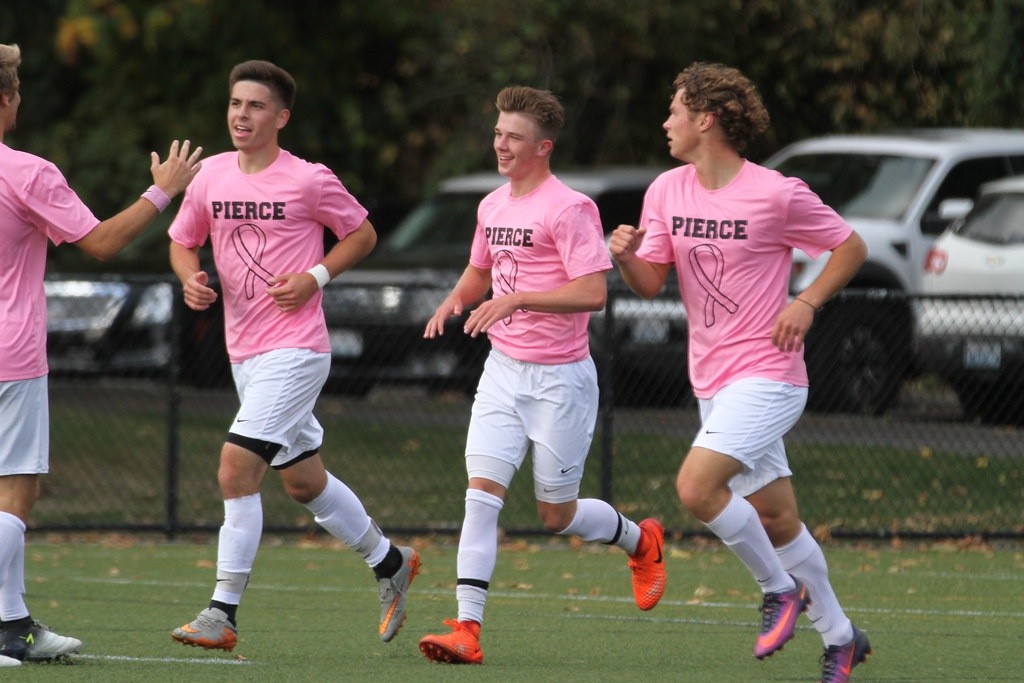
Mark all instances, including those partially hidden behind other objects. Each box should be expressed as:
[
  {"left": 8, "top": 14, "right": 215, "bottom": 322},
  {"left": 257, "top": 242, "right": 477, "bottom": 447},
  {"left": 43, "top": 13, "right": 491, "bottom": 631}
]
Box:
[
  {"left": 319, "top": 165, "right": 676, "bottom": 402},
  {"left": 587, "top": 125, "right": 1024, "bottom": 417}
]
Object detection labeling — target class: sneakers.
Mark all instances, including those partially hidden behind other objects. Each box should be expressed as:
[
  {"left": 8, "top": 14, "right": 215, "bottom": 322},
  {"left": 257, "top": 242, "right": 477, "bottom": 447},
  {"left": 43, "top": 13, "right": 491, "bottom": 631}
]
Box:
[
  {"left": 819, "top": 625, "right": 872, "bottom": 683},
  {"left": 168, "top": 607, "right": 239, "bottom": 653},
  {"left": 417, "top": 620, "right": 483, "bottom": 665},
  {"left": 752, "top": 572, "right": 812, "bottom": 660},
  {"left": 627, "top": 517, "right": 667, "bottom": 611},
  {"left": 0, "top": 619, "right": 82, "bottom": 666},
  {"left": 375, "top": 544, "right": 423, "bottom": 643}
]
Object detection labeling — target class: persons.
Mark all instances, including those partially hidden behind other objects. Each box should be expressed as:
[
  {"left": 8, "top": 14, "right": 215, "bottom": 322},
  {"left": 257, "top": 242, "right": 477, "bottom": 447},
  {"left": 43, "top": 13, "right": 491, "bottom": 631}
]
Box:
[
  {"left": 608, "top": 63, "right": 875, "bottom": 682},
  {"left": 0, "top": 41, "right": 203, "bottom": 671},
  {"left": 419, "top": 85, "right": 666, "bottom": 674},
  {"left": 166, "top": 59, "right": 420, "bottom": 655}
]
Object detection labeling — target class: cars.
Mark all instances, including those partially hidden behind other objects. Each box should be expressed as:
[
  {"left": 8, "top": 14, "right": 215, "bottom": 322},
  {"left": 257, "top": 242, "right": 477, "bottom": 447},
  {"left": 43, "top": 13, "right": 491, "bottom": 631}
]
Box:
[
  {"left": 916, "top": 174, "right": 1024, "bottom": 423},
  {"left": 42, "top": 222, "right": 236, "bottom": 389}
]
